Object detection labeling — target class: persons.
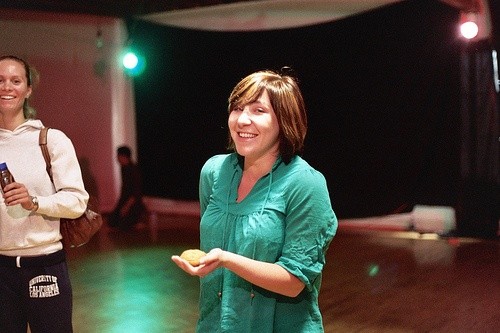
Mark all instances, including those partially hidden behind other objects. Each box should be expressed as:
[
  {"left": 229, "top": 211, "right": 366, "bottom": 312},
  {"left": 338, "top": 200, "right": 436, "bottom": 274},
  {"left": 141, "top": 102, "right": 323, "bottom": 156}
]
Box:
[
  {"left": 1, "top": 55, "right": 90, "bottom": 333},
  {"left": 107, "top": 145, "right": 153, "bottom": 230},
  {"left": 169, "top": 69, "right": 338, "bottom": 333}
]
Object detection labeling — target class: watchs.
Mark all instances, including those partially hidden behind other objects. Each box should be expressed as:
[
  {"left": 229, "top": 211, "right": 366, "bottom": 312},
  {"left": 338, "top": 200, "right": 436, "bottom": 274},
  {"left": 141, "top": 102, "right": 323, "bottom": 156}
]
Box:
[{"left": 27, "top": 195, "right": 38, "bottom": 212}]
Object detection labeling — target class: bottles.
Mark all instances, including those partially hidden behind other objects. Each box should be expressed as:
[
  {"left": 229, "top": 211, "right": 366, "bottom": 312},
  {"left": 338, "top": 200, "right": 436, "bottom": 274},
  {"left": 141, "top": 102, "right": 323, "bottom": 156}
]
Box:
[{"left": 0, "top": 162, "right": 16, "bottom": 203}]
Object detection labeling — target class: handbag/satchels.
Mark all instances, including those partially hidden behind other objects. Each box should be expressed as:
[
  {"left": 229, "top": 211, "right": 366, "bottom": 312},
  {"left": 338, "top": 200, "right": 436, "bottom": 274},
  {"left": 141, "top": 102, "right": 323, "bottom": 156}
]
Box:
[{"left": 39, "top": 126, "right": 102, "bottom": 248}]
